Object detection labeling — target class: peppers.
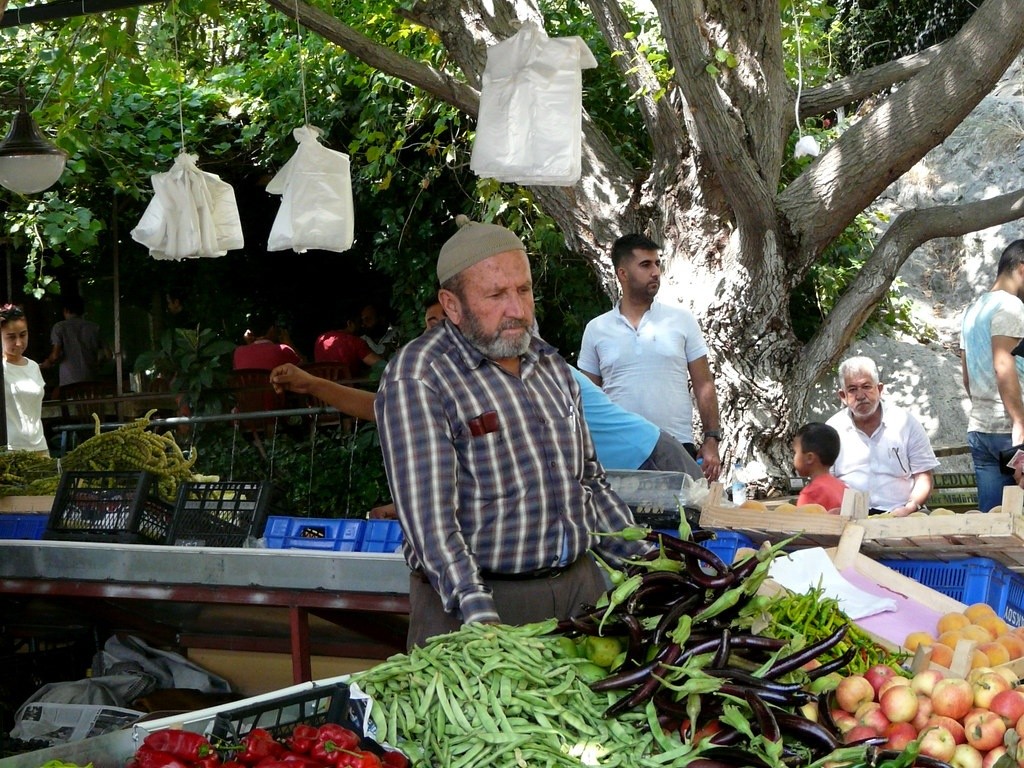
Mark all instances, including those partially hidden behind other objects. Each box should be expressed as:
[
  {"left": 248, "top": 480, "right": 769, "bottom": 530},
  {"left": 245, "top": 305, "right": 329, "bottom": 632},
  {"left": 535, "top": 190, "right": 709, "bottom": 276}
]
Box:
[
  {"left": 40, "top": 724, "right": 409, "bottom": 768},
  {"left": 754, "top": 572, "right": 916, "bottom": 679}
]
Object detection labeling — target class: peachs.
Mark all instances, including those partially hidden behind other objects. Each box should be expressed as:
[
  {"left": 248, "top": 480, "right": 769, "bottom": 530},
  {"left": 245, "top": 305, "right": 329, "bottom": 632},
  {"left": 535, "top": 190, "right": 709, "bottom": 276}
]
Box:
[{"left": 902, "top": 601, "right": 1024, "bottom": 673}]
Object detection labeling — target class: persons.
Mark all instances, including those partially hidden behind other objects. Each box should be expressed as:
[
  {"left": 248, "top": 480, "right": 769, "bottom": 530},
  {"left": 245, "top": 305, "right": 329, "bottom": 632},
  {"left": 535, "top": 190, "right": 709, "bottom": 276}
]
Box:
[
  {"left": 39, "top": 295, "right": 114, "bottom": 398},
  {"left": 0, "top": 303, "right": 48, "bottom": 451},
  {"left": 792, "top": 422, "right": 848, "bottom": 511},
  {"left": 314, "top": 313, "right": 382, "bottom": 377},
  {"left": 372, "top": 214, "right": 658, "bottom": 652},
  {"left": 576, "top": 234, "right": 721, "bottom": 488},
  {"left": 826, "top": 356, "right": 941, "bottom": 517},
  {"left": 960, "top": 238, "right": 1024, "bottom": 513},
  {"left": 268, "top": 296, "right": 704, "bottom": 520},
  {"left": 166, "top": 287, "right": 196, "bottom": 340},
  {"left": 233, "top": 307, "right": 305, "bottom": 371}
]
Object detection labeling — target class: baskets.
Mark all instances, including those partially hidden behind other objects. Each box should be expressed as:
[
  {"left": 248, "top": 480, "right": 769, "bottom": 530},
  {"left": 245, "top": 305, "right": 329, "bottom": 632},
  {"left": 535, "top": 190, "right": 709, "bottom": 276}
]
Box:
[
  {"left": 651, "top": 528, "right": 755, "bottom": 566},
  {"left": 882, "top": 553, "right": 1024, "bottom": 626}
]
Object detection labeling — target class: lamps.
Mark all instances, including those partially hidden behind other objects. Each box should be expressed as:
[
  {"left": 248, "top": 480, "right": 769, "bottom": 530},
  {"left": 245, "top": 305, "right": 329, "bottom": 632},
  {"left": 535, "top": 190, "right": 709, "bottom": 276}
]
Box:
[{"left": 0, "top": 78, "right": 69, "bottom": 195}]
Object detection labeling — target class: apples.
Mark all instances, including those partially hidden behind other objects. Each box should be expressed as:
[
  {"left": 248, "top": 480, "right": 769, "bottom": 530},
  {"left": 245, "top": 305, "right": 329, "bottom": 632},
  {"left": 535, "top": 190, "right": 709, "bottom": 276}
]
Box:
[{"left": 801, "top": 656, "right": 1024, "bottom": 768}]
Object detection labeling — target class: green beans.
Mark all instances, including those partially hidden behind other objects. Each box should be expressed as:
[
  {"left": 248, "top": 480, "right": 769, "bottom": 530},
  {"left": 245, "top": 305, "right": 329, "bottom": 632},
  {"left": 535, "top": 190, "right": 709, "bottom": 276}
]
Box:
[{"left": 343, "top": 622, "right": 699, "bottom": 768}]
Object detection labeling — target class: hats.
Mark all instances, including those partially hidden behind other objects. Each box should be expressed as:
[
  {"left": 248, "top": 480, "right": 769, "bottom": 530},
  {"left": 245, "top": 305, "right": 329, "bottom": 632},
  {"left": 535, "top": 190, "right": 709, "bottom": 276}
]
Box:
[{"left": 436, "top": 212, "right": 526, "bottom": 286}]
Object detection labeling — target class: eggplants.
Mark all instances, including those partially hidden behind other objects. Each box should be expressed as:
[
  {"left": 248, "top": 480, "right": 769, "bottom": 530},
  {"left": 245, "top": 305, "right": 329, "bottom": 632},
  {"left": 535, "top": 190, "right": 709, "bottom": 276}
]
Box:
[
  {"left": 548, "top": 614, "right": 957, "bottom": 768},
  {"left": 551, "top": 494, "right": 806, "bottom": 647}
]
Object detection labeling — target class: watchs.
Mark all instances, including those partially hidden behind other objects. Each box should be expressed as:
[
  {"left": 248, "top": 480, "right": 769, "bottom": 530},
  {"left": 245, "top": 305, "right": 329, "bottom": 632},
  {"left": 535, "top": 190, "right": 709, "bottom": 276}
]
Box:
[{"left": 703, "top": 431, "right": 722, "bottom": 442}]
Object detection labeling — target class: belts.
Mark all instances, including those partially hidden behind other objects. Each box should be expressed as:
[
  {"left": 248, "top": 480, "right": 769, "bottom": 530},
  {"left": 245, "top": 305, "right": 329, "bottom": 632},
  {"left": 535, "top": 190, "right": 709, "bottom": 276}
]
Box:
[{"left": 479, "top": 563, "right": 571, "bottom": 581}]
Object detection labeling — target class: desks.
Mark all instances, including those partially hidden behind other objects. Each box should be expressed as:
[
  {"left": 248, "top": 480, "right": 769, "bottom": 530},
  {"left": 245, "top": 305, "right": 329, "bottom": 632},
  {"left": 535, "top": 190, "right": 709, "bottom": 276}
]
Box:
[{"left": 40, "top": 390, "right": 192, "bottom": 424}]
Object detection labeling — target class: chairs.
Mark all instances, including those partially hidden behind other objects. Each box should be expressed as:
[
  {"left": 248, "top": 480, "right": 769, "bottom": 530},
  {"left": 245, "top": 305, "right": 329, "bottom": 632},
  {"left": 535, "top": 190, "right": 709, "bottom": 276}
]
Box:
[
  {"left": 59, "top": 377, "right": 123, "bottom": 456},
  {"left": 295, "top": 361, "right": 365, "bottom": 440},
  {"left": 230, "top": 366, "right": 296, "bottom": 441}
]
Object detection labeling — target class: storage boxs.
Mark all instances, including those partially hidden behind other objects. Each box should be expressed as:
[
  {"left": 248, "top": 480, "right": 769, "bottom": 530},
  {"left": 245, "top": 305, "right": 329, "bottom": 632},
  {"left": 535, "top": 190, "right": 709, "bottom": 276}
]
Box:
[
  {"left": 42, "top": 468, "right": 179, "bottom": 546},
  {"left": 209, "top": 682, "right": 413, "bottom": 768},
  {"left": 646, "top": 529, "right": 761, "bottom": 569},
  {"left": 0, "top": 514, "right": 50, "bottom": 540},
  {"left": 874, "top": 556, "right": 1023, "bottom": 629},
  {"left": 262, "top": 515, "right": 407, "bottom": 554},
  {"left": 163, "top": 480, "right": 294, "bottom": 548}
]
